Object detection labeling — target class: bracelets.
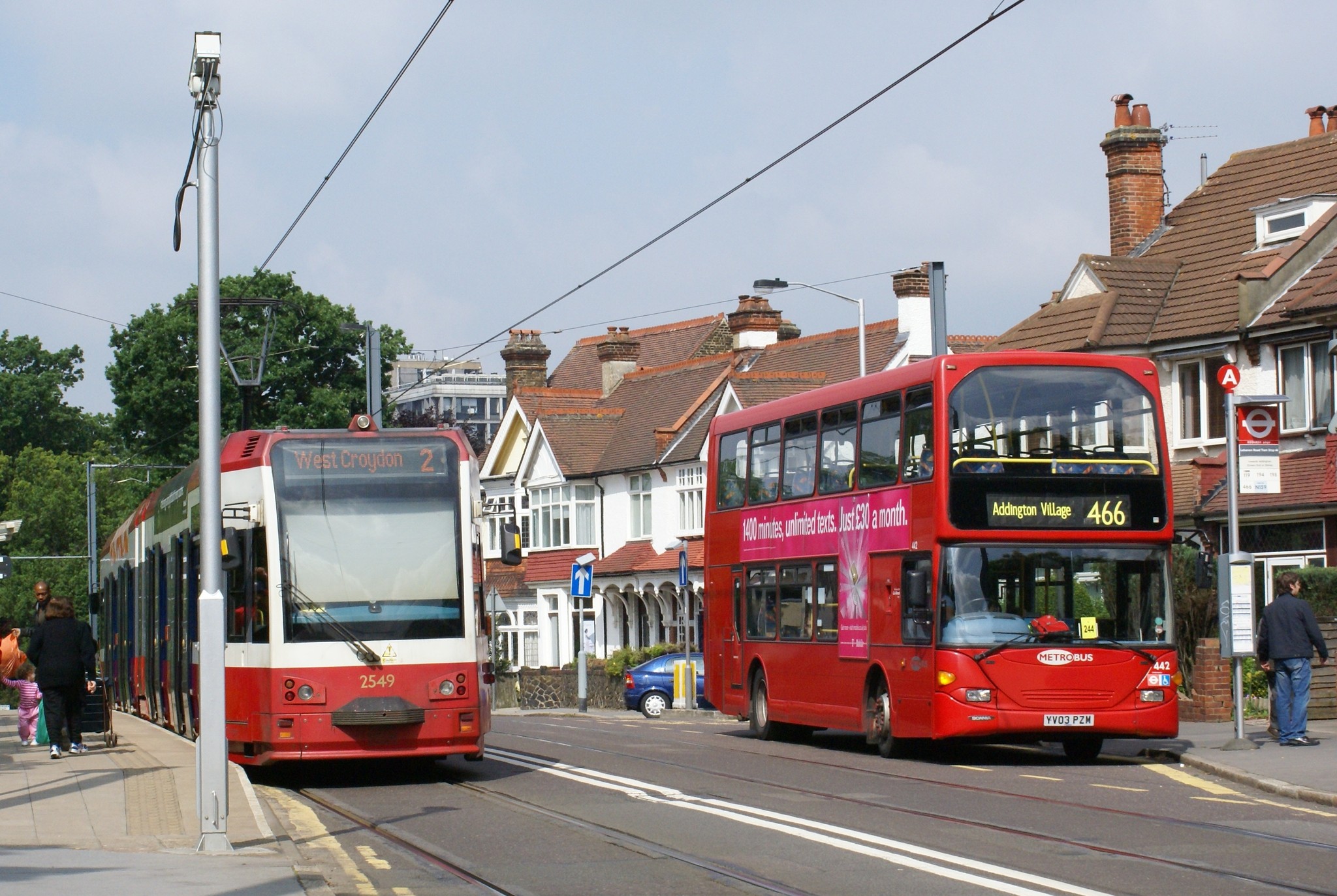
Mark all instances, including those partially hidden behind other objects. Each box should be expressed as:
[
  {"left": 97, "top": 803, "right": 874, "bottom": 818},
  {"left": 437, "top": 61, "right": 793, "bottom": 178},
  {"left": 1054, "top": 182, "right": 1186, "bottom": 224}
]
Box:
[{"left": 263, "top": 573, "right": 267, "bottom": 582}]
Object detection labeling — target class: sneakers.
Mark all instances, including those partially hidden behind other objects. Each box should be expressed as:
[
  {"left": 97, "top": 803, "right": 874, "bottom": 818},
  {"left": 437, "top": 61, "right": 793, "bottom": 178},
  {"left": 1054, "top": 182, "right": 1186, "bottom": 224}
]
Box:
[
  {"left": 50, "top": 745, "right": 62, "bottom": 758},
  {"left": 69, "top": 742, "right": 88, "bottom": 753}
]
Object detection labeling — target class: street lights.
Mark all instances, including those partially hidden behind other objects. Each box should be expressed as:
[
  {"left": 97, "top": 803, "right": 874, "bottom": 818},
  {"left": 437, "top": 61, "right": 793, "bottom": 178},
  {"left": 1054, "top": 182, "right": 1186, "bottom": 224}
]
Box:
[{"left": 752, "top": 278, "right": 867, "bottom": 378}]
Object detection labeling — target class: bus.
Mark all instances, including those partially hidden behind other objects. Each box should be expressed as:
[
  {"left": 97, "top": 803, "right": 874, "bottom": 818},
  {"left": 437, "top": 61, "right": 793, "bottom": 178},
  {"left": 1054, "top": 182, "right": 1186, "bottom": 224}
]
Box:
[
  {"left": 97, "top": 294, "right": 525, "bottom": 777},
  {"left": 701, "top": 350, "right": 1183, "bottom": 758}
]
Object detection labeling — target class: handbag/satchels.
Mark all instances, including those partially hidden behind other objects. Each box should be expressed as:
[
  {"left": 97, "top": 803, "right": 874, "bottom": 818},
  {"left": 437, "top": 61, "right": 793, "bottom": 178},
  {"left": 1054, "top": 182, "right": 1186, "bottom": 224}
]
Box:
[
  {"left": 0, "top": 633, "right": 27, "bottom": 680},
  {"left": 36, "top": 696, "right": 50, "bottom": 744}
]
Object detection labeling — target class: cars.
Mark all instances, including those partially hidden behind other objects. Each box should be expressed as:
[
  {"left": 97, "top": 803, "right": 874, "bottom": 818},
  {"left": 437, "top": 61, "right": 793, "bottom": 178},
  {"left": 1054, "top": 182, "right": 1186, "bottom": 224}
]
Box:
[{"left": 624, "top": 650, "right": 710, "bottom": 718}]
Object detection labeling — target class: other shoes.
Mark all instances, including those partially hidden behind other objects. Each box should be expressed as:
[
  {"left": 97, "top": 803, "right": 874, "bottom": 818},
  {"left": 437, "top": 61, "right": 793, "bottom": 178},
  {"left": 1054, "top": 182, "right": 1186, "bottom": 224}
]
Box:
[
  {"left": 30, "top": 739, "right": 40, "bottom": 745},
  {"left": 22, "top": 739, "right": 29, "bottom": 746},
  {"left": 1267, "top": 727, "right": 1279, "bottom": 738}
]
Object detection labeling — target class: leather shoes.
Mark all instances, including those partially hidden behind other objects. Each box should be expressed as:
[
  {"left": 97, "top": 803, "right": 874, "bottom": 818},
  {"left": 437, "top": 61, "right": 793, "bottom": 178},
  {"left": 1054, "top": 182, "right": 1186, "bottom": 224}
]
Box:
[
  {"left": 1290, "top": 737, "right": 1320, "bottom": 745},
  {"left": 1280, "top": 740, "right": 1291, "bottom": 746}
]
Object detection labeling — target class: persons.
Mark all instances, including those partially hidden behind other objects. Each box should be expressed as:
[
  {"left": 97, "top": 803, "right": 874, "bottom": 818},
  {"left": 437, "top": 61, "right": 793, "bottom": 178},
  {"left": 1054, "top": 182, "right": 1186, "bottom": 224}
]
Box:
[
  {"left": 1256, "top": 618, "right": 1281, "bottom": 738},
  {"left": 28, "top": 596, "right": 97, "bottom": 756},
  {"left": 942, "top": 579, "right": 996, "bottom": 620},
  {"left": 11, "top": 581, "right": 52, "bottom": 640},
  {"left": 254, "top": 567, "right": 268, "bottom": 582},
  {"left": 1258, "top": 573, "right": 1329, "bottom": 746},
  {"left": 0, "top": 664, "right": 43, "bottom": 746}
]
